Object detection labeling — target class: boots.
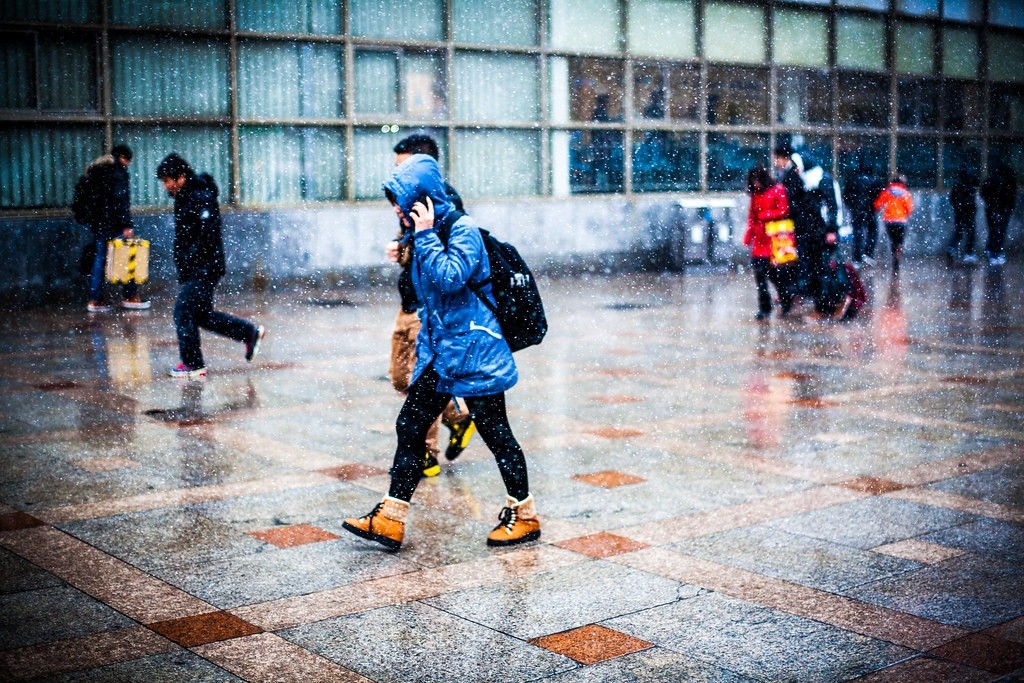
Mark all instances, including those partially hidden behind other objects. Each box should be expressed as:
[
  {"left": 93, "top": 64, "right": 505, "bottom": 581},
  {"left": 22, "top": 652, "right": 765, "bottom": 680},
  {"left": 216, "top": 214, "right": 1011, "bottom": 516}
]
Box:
[
  {"left": 343, "top": 493, "right": 410, "bottom": 551},
  {"left": 487, "top": 492, "right": 541, "bottom": 547}
]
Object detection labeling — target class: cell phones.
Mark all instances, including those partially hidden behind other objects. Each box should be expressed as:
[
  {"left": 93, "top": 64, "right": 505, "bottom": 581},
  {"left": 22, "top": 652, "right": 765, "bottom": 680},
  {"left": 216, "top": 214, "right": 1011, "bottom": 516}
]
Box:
[{"left": 408, "top": 195, "right": 434, "bottom": 225}]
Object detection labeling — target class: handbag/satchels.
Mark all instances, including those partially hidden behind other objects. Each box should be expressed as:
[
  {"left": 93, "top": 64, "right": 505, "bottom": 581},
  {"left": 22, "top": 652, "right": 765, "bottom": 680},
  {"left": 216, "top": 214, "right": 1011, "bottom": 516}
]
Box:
[
  {"left": 766, "top": 210, "right": 799, "bottom": 267},
  {"left": 817, "top": 240, "right": 853, "bottom": 304},
  {"left": 81, "top": 238, "right": 97, "bottom": 270},
  {"left": 104, "top": 233, "right": 150, "bottom": 284}
]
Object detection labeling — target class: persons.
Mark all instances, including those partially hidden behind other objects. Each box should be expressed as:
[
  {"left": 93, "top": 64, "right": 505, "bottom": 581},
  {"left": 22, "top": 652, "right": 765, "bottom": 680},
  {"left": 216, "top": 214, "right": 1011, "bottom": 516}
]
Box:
[
  {"left": 843, "top": 169, "right": 913, "bottom": 269},
  {"left": 87, "top": 144, "right": 151, "bottom": 312},
  {"left": 945, "top": 164, "right": 1016, "bottom": 266},
  {"left": 743, "top": 144, "right": 855, "bottom": 320},
  {"left": 156, "top": 154, "right": 265, "bottom": 378},
  {"left": 387, "top": 134, "right": 476, "bottom": 477},
  {"left": 342, "top": 153, "right": 541, "bottom": 551}
]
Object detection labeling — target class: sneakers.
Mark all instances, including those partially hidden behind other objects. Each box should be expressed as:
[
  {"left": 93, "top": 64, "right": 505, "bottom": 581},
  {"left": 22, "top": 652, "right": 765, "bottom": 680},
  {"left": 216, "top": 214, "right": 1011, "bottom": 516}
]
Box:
[
  {"left": 420, "top": 452, "right": 441, "bottom": 477},
  {"left": 121, "top": 292, "right": 150, "bottom": 308},
  {"left": 169, "top": 363, "right": 208, "bottom": 378},
  {"left": 242, "top": 324, "right": 265, "bottom": 362},
  {"left": 441, "top": 414, "right": 477, "bottom": 460},
  {"left": 86, "top": 298, "right": 113, "bottom": 313}
]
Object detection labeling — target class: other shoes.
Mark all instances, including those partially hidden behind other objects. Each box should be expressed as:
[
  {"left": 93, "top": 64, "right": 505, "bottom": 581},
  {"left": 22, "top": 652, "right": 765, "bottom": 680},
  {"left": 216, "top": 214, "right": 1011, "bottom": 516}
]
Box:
[
  {"left": 944, "top": 245, "right": 1007, "bottom": 268},
  {"left": 755, "top": 291, "right": 851, "bottom": 323},
  {"left": 892, "top": 245, "right": 903, "bottom": 270},
  {"left": 850, "top": 254, "right": 876, "bottom": 272}
]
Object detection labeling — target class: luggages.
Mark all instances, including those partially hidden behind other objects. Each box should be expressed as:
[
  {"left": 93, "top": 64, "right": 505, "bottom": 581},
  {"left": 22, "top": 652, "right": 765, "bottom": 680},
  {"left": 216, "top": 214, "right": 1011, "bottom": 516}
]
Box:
[{"left": 827, "top": 259, "right": 866, "bottom": 317}]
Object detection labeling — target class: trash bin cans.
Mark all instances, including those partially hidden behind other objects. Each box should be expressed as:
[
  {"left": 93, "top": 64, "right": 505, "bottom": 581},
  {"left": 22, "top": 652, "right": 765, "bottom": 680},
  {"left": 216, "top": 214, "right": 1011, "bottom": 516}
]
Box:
[{"left": 670, "top": 198, "right": 736, "bottom": 277}]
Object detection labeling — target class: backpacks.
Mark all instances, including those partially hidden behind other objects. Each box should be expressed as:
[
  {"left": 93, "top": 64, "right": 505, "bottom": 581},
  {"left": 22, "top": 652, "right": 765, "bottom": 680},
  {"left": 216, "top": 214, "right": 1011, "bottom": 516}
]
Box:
[
  {"left": 72, "top": 164, "right": 124, "bottom": 225},
  {"left": 440, "top": 209, "right": 547, "bottom": 352}
]
